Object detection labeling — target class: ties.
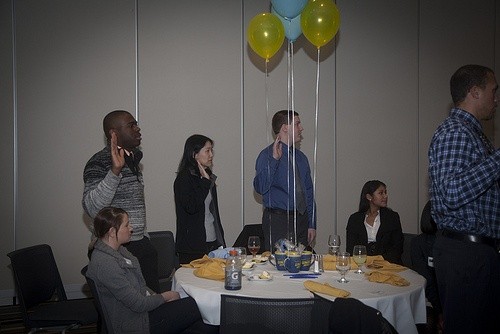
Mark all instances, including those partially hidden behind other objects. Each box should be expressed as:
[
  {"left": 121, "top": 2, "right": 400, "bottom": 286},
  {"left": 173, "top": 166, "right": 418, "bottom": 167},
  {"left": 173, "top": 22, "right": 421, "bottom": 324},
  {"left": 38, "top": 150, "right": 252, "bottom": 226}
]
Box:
[{"left": 290, "top": 149, "right": 306, "bottom": 215}]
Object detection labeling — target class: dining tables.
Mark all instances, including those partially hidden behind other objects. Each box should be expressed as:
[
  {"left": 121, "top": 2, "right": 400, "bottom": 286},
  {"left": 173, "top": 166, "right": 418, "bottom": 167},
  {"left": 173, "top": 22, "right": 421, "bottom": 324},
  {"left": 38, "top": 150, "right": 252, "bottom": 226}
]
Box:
[{"left": 169, "top": 252, "right": 429, "bottom": 334}]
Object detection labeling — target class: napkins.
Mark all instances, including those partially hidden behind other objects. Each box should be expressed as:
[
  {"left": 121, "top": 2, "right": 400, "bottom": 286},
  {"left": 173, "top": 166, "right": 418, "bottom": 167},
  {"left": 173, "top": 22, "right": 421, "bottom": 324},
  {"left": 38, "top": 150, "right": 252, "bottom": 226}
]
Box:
[{"left": 180, "top": 245, "right": 411, "bottom": 299}]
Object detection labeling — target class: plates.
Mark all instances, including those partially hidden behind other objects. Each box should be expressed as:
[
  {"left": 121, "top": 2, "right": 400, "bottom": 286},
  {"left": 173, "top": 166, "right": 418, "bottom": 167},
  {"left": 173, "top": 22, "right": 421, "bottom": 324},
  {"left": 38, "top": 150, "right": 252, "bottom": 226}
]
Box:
[{"left": 246, "top": 274, "right": 271, "bottom": 281}]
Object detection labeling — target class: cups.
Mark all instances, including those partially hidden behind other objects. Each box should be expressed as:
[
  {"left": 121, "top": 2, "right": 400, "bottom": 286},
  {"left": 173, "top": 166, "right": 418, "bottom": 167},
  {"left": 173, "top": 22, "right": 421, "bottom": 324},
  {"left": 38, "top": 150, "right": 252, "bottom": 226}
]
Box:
[
  {"left": 268, "top": 250, "right": 285, "bottom": 270},
  {"left": 301, "top": 251, "right": 316, "bottom": 271},
  {"left": 284, "top": 253, "right": 301, "bottom": 273}
]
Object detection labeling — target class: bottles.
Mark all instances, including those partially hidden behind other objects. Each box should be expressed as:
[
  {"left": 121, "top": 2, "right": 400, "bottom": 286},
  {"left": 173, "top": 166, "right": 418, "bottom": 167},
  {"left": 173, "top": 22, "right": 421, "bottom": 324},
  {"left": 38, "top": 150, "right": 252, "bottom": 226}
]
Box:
[{"left": 224, "top": 250, "right": 241, "bottom": 290}]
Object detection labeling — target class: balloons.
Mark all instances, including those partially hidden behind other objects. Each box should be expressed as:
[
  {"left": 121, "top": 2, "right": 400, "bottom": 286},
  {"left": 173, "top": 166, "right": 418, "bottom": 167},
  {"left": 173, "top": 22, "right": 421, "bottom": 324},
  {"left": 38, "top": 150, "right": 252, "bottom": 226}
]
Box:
[
  {"left": 301, "top": 0, "right": 341, "bottom": 49},
  {"left": 271, "top": 0, "right": 309, "bottom": 22},
  {"left": 270, "top": 7, "right": 303, "bottom": 43},
  {"left": 246, "top": 13, "right": 286, "bottom": 63}
]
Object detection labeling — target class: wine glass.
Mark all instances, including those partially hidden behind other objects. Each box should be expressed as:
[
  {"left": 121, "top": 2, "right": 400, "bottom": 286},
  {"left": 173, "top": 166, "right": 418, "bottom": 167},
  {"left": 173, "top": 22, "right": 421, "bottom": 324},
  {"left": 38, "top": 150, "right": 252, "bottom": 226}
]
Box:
[
  {"left": 353, "top": 245, "right": 367, "bottom": 275},
  {"left": 335, "top": 252, "right": 351, "bottom": 284},
  {"left": 328, "top": 235, "right": 340, "bottom": 255},
  {"left": 248, "top": 236, "right": 260, "bottom": 259}
]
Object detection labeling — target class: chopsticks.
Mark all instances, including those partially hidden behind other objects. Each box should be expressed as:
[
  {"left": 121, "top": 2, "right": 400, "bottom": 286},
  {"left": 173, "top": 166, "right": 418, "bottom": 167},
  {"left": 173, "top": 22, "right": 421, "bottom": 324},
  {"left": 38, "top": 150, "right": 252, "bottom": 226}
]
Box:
[{"left": 283, "top": 273, "right": 322, "bottom": 278}]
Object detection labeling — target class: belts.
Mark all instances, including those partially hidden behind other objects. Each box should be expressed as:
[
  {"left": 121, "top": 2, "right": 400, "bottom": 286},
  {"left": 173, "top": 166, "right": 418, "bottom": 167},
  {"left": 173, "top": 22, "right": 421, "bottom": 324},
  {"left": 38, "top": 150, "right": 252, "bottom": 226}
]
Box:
[{"left": 436, "top": 229, "right": 500, "bottom": 247}]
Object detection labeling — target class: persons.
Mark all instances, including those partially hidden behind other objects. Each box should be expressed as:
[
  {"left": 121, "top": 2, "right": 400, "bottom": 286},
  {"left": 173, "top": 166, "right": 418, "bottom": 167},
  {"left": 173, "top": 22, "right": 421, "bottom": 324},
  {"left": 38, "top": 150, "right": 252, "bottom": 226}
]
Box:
[
  {"left": 346, "top": 179, "right": 404, "bottom": 264},
  {"left": 420, "top": 200, "right": 437, "bottom": 240},
  {"left": 85, "top": 206, "right": 205, "bottom": 334},
  {"left": 426, "top": 64, "right": 500, "bottom": 334},
  {"left": 253, "top": 109, "right": 317, "bottom": 256},
  {"left": 172, "top": 134, "right": 226, "bottom": 264},
  {"left": 80, "top": 109, "right": 173, "bottom": 292}
]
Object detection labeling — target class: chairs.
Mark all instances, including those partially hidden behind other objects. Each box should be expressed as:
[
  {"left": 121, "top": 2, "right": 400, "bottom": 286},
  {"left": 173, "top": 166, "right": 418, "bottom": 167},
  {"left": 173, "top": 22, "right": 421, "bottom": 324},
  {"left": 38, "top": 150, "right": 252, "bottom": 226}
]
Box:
[
  {"left": 80, "top": 264, "right": 110, "bottom": 334},
  {"left": 6, "top": 243, "right": 101, "bottom": 334},
  {"left": 400, "top": 233, "right": 418, "bottom": 266},
  {"left": 144, "top": 231, "right": 175, "bottom": 293},
  {"left": 234, "top": 225, "right": 265, "bottom": 255},
  {"left": 220, "top": 294, "right": 314, "bottom": 334},
  {"left": 311, "top": 290, "right": 399, "bottom": 334}
]
